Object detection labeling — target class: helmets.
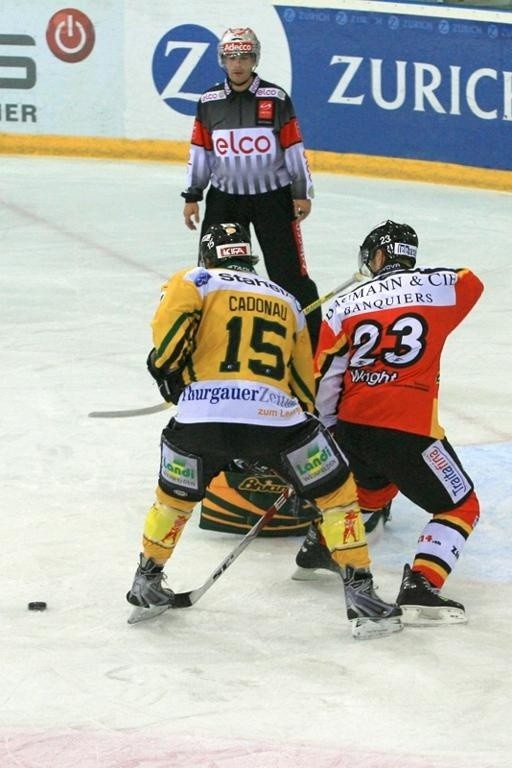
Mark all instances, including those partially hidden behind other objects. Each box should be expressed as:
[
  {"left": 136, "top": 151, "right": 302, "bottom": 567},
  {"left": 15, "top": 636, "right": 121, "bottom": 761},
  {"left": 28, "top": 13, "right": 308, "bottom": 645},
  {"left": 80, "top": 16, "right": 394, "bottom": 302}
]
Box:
[
  {"left": 202, "top": 222, "right": 260, "bottom": 266},
  {"left": 359, "top": 219, "right": 418, "bottom": 270},
  {"left": 218, "top": 27, "right": 261, "bottom": 67}
]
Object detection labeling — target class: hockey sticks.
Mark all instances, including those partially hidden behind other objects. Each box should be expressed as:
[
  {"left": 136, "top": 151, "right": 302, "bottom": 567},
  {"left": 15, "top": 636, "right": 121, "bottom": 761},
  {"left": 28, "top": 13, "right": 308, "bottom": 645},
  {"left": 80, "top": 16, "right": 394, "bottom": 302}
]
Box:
[
  {"left": 89, "top": 271, "right": 364, "bottom": 419},
  {"left": 164, "top": 484, "right": 293, "bottom": 609}
]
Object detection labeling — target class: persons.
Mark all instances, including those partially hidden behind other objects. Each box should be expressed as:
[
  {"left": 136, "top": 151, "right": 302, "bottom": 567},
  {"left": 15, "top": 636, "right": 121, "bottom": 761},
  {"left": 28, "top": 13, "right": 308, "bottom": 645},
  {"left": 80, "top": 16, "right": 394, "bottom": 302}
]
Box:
[
  {"left": 126, "top": 222, "right": 403, "bottom": 622},
  {"left": 295, "top": 220, "right": 484, "bottom": 613},
  {"left": 183, "top": 28, "right": 321, "bottom": 358}
]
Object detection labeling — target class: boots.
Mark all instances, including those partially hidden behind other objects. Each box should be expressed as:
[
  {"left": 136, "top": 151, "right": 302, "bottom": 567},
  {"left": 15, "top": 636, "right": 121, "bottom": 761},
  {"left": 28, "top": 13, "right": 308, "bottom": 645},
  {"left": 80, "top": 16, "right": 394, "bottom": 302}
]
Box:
[
  {"left": 126, "top": 552, "right": 176, "bottom": 606},
  {"left": 296, "top": 517, "right": 338, "bottom": 570},
  {"left": 396, "top": 564, "right": 465, "bottom": 611},
  {"left": 337, "top": 564, "right": 403, "bottom": 622}
]
